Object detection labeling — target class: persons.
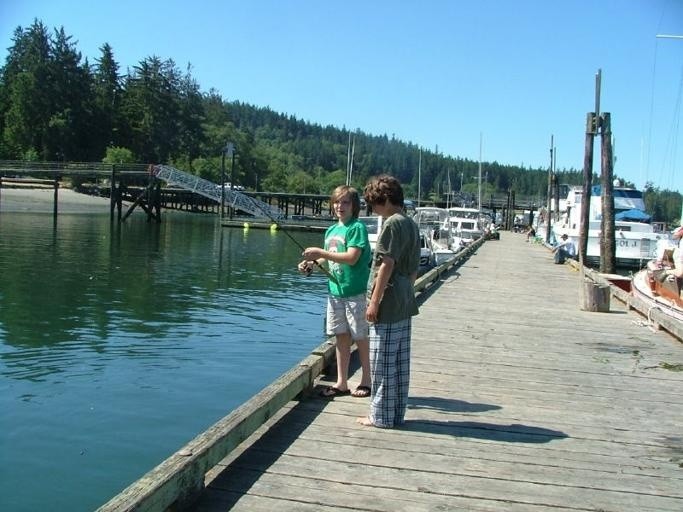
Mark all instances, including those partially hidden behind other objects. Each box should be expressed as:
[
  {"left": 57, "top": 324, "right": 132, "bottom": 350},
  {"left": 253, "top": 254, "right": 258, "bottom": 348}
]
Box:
[
  {"left": 356, "top": 174, "right": 420, "bottom": 429},
  {"left": 653, "top": 248, "right": 682, "bottom": 282},
  {"left": 490, "top": 223, "right": 500, "bottom": 240},
  {"left": 524, "top": 225, "right": 534, "bottom": 242},
  {"left": 484, "top": 224, "right": 491, "bottom": 235},
  {"left": 298, "top": 185, "right": 371, "bottom": 397},
  {"left": 550, "top": 234, "right": 576, "bottom": 263}
]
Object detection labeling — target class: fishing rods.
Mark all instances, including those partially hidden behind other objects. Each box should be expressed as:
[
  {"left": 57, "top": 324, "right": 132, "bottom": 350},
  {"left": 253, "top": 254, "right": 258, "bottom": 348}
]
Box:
[{"left": 198, "top": 146, "right": 339, "bottom": 285}]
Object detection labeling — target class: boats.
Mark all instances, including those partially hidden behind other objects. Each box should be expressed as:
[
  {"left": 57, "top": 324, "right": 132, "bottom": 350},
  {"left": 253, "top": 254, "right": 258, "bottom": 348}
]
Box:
[
  {"left": 529, "top": 181, "right": 683, "bottom": 322},
  {"left": 358, "top": 205, "right": 490, "bottom": 278}
]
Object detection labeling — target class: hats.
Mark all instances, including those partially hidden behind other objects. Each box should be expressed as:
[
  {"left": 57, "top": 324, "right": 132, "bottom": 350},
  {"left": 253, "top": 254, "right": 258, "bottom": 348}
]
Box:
[{"left": 561, "top": 234, "right": 568, "bottom": 238}]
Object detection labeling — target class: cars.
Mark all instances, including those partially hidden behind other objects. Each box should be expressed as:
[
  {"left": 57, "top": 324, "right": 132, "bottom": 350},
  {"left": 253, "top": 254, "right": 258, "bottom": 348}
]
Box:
[{"left": 216, "top": 182, "right": 244, "bottom": 191}]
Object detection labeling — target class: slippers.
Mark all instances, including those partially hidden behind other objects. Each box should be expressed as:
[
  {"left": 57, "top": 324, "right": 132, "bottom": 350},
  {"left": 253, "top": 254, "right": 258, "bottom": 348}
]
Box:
[
  {"left": 320, "top": 386, "right": 350, "bottom": 397},
  {"left": 351, "top": 386, "right": 371, "bottom": 397}
]
